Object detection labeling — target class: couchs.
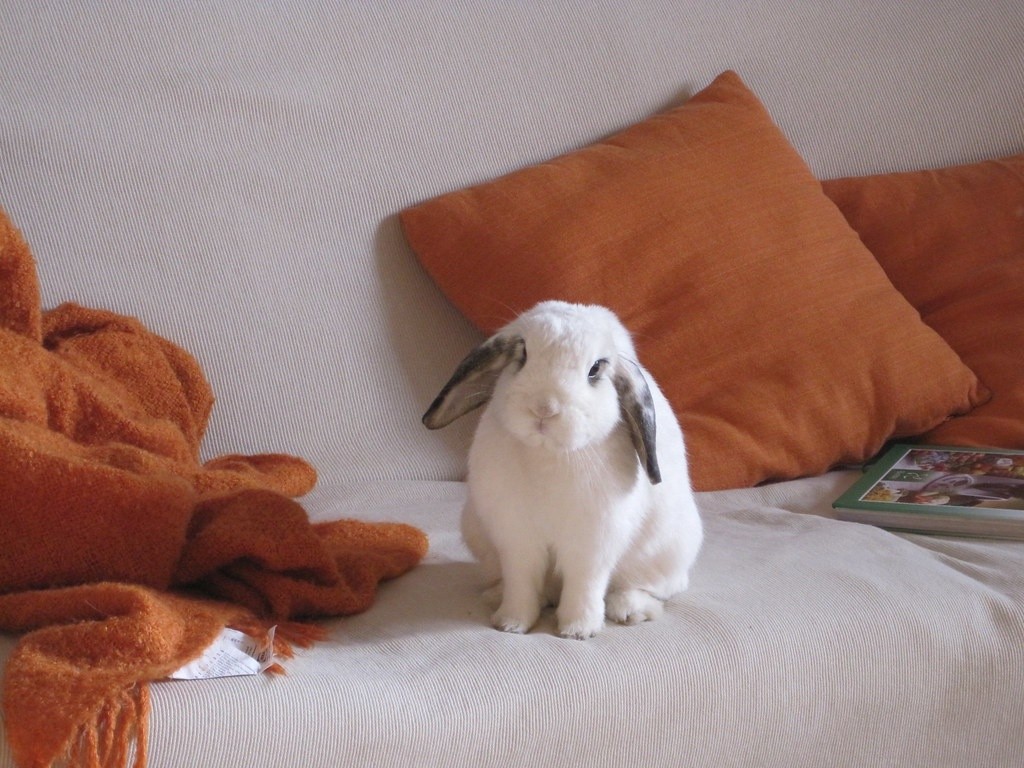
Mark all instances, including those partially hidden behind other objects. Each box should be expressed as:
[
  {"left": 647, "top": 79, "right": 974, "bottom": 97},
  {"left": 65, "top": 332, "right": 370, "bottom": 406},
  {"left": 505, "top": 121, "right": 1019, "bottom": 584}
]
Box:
[{"left": 0, "top": 0, "right": 1024, "bottom": 768}]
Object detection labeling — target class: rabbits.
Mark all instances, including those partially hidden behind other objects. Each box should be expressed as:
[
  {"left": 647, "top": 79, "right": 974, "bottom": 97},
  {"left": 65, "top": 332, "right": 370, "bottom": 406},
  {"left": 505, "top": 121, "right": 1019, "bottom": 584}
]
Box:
[{"left": 421, "top": 299, "right": 703, "bottom": 638}]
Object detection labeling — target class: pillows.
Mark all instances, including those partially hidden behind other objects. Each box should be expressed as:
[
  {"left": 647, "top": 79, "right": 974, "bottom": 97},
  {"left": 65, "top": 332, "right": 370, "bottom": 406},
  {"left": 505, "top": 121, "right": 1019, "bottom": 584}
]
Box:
[
  {"left": 399, "top": 70, "right": 991, "bottom": 500},
  {"left": 819, "top": 151, "right": 1024, "bottom": 451}
]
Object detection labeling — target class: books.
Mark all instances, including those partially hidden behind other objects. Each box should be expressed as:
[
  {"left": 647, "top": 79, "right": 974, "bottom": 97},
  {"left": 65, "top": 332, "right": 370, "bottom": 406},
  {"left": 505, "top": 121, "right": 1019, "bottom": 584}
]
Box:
[{"left": 831, "top": 441, "right": 1024, "bottom": 542}]
